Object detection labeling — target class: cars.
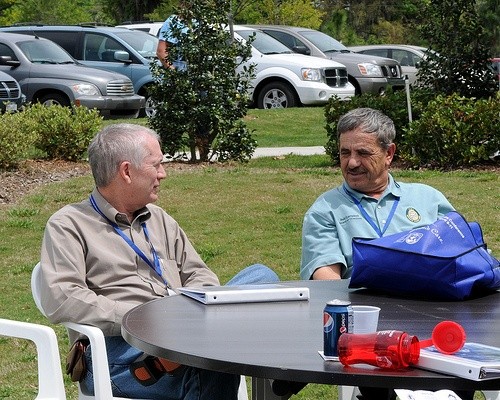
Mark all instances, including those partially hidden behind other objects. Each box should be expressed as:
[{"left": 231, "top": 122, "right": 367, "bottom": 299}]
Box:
[
  {"left": 347, "top": 43, "right": 500, "bottom": 98},
  {"left": 0, "top": 70, "right": 28, "bottom": 117}
]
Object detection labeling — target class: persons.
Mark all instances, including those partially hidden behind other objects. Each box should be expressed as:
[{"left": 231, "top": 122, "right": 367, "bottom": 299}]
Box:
[
  {"left": 35, "top": 122, "right": 310, "bottom": 400},
  {"left": 298, "top": 108, "right": 492, "bottom": 281},
  {"left": 155, "top": 10, "right": 208, "bottom": 100}
]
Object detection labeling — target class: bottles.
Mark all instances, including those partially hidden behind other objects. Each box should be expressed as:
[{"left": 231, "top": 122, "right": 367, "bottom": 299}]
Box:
[{"left": 337, "top": 320, "right": 466, "bottom": 371}]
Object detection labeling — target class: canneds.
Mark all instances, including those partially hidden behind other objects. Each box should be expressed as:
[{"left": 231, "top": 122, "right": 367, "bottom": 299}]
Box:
[{"left": 323, "top": 298, "right": 354, "bottom": 356}]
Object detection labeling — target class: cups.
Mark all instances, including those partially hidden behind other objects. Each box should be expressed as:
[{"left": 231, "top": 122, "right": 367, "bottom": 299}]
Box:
[{"left": 347, "top": 305, "right": 382, "bottom": 335}]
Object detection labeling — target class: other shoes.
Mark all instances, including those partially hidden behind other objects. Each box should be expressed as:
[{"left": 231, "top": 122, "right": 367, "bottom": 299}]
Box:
[{"left": 131, "top": 352, "right": 185, "bottom": 387}]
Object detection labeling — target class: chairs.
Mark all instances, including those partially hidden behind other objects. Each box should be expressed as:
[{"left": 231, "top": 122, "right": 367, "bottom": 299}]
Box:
[
  {"left": 401, "top": 56, "right": 408, "bottom": 65},
  {"left": 0, "top": 262, "right": 249, "bottom": 400}
]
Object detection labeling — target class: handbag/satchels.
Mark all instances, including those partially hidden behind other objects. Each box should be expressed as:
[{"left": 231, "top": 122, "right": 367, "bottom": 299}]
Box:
[{"left": 348, "top": 211, "right": 500, "bottom": 301}]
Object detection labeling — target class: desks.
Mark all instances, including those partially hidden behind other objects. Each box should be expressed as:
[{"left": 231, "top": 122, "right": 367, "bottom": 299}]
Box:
[{"left": 121, "top": 279, "right": 500, "bottom": 400}]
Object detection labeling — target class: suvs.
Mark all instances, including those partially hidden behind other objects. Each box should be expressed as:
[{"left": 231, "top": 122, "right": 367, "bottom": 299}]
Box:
[
  {"left": 0, "top": 30, "right": 147, "bottom": 121},
  {"left": 240, "top": 24, "right": 406, "bottom": 100},
  {"left": 0, "top": 24, "right": 209, "bottom": 122},
  {"left": 98, "top": 20, "right": 356, "bottom": 110}
]
